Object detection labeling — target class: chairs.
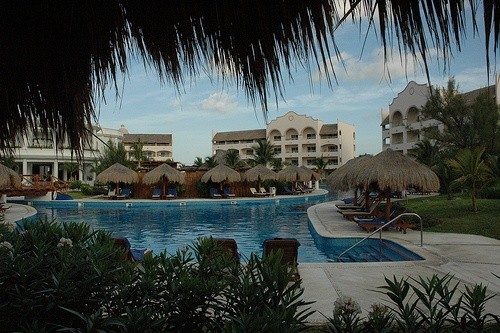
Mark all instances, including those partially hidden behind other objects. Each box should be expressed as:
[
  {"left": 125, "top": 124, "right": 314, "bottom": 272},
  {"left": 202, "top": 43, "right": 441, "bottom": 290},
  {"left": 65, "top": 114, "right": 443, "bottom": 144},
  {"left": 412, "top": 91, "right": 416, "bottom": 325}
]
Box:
[
  {"left": 210, "top": 187, "right": 222, "bottom": 198},
  {"left": 224, "top": 187, "right": 235, "bottom": 199},
  {"left": 250, "top": 187, "right": 274, "bottom": 198},
  {"left": 117, "top": 187, "right": 130, "bottom": 199},
  {"left": 109, "top": 237, "right": 154, "bottom": 269},
  {"left": 216, "top": 238, "right": 242, "bottom": 270},
  {"left": 152, "top": 188, "right": 161, "bottom": 200},
  {"left": 104, "top": 188, "right": 116, "bottom": 200},
  {"left": 335, "top": 192, "right": 429, "bottom": 234},
  {"left": 285, "top": 183, "right": 314, "bottom": 196},
  {"left": 166, "top": 185, "right": 177, "bottom": 199},
  {"left": 262, "top": 236, "right": 302, "bottom": 287}
]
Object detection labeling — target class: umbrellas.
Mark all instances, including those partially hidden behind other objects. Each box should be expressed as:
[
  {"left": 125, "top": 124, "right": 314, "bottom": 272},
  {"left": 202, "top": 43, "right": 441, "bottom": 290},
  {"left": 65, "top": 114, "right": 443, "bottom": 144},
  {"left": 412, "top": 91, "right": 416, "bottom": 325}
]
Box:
[
  {"left": 98, "top": 163, "right": 139, "bottom": 194},
  {"left": 243, "top": 163, "right": 322, "bottom": 191},
  {"left": 326, "top": 149, "right": 440, "bottom": 221},
  {"left": 143, "top": 163, "right": 186, "bottom": 196},
  {"left": 0, "top": 163, "right": 21, "bottom": 188},
  {"left": 202, "top": 163, "right": 241, "bottom": 194}
]
